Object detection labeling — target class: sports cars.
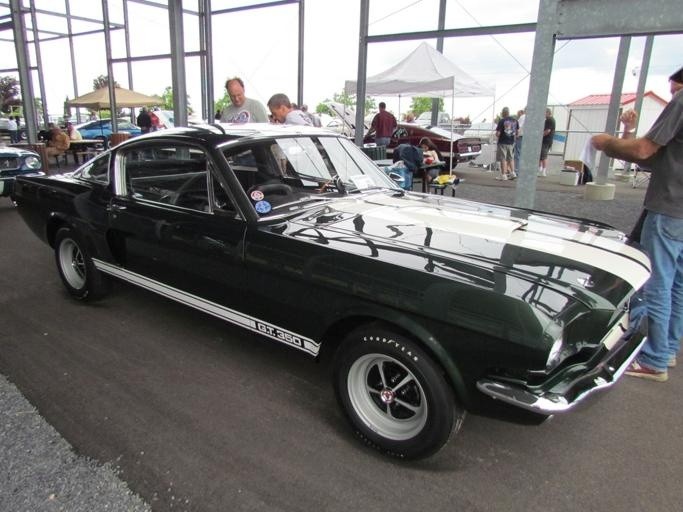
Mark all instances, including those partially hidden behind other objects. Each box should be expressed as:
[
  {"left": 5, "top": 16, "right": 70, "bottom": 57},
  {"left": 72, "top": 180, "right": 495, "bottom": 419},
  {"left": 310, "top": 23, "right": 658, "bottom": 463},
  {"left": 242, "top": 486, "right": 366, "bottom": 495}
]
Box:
[{"left": 11, "top": 122, "right": 652, "bottom": 464}]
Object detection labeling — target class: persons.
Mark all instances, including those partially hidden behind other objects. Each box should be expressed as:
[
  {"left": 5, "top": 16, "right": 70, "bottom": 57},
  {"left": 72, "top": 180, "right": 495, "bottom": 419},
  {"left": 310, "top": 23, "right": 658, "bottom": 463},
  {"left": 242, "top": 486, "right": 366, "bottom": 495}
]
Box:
[
  {"left": 215, "top": 78, "right": 322, "bottom": 127},
  {"left": 495, "top": 106, "right": 556, "bottom": 182},
  {"left": 92, "top": 111, "right": 97, "bottom": 120},
  {"left": 367, "top": 102, "right": 397, "bottom": 148},
  {"left": 137, "top": 105, "right": 166, "bottom": 134},
  {"left": 8, "top": 116, "right": 21, "bottom": 144},
  {"left": 419, "top": 137, "right": 442, "bottom": 194},
  {"left": 45, "top": 122, "right": 81, "bottom": 169},
  {"left": 590, "top": 91, "right": 683, "bottom": 383},
  {"left": 621, "top": 69, "right": 683, "bottom": 241}
]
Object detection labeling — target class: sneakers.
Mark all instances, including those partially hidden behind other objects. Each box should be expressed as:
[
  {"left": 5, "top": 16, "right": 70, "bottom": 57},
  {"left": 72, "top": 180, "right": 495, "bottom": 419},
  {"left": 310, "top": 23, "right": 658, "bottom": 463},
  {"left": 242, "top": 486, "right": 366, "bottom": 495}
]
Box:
[
  {"left": 624, "top": 358, "right": 669, "bottom": 383},
  {"left": 496, "top": 173, "right": 508, "bottom": 181},
  {"left": 507, "top": 174, "right": 516, "bottom": 180}
]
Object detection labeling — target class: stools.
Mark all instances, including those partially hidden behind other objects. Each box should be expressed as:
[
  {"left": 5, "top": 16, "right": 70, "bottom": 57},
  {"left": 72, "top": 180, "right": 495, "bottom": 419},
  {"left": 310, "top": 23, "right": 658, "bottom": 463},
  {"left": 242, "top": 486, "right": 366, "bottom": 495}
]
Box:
[{"left": 55, "top": 153, "right": 68, "bottom": 168}]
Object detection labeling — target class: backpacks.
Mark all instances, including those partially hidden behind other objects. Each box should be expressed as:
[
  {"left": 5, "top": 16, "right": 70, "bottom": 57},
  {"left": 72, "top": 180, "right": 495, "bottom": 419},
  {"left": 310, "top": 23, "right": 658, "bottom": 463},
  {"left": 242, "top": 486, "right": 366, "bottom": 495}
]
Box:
[{"left": 57, "top": 132, "right": 69, "bottom": 151}]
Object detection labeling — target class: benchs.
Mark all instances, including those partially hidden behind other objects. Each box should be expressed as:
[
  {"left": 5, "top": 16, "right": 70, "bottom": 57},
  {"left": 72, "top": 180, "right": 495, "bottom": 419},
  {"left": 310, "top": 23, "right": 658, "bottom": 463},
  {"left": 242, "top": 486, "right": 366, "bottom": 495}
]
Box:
[
  {"left": 428, "top": 175, "right": 465, "bottom": 197},
  {"left": 76, "top": 151, "right": 100, "bottom": 166}
]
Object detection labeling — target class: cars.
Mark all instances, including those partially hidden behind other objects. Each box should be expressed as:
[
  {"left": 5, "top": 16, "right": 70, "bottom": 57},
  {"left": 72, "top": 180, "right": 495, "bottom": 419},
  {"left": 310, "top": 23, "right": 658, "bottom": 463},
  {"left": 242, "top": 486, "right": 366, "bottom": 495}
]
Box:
[
  {"left": 53, "top": 117, "right": 142, "bottom": 148},
  {"left": 150, "top": 110, "right": 174, "bottom": 129},
  {"left": 314, "top": 113, "right": 337, "bottom": 126},
  {"left": 321, "top": 100, "right": 483, "bottom": 171},
  {"left": 462, "top": 121, "right": 498, "bottom": 146},
  {"left": 0, "top": 118, "right": 12, "bottom": 134},
  {"left": 17, "top": 123, "right": 42, "bottom": 139},
  {"left": 0, "top": 141, "right": 43, "bottom": 199},
  {"left": 414, "top": 109, "right": 451, "bottom": 127}
]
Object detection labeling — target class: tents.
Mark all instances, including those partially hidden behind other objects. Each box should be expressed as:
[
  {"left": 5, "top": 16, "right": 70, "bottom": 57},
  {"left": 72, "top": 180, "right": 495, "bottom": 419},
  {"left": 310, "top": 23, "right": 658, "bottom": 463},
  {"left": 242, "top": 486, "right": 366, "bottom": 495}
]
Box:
[
  {"left": 344, "top": 41, "right": 496, "bottom": 176},
  {"left": 63, "top": 86, "right": 164, "bottom": 141}
]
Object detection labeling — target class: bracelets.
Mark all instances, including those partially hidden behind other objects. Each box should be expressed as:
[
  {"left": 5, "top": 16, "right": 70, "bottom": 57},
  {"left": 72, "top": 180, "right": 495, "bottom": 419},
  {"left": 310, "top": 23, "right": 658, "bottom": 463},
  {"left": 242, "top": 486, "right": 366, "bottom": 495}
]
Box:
[{"left": 623, "top": 125, "right": 637, "bottom": 136}]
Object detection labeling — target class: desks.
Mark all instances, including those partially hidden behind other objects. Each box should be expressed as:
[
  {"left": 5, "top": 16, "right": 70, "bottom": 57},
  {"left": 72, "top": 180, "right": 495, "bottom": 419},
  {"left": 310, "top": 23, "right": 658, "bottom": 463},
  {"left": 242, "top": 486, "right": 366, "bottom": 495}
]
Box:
[
  {"left": 65, "top": 140, "right": 104, "bottom": 166},
  {"left": 374, "top": 157, "right": 446, "bottom": 193}
]
